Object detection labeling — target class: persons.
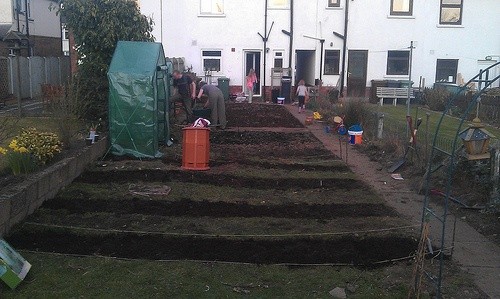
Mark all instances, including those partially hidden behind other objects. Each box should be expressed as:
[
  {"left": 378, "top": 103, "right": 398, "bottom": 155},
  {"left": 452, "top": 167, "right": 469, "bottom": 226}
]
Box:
[
  {"left": 172, "top": 70, "right": 196, "bottom": 111},
  {"left": 247, "top": 68, "right": 258, "bottom": 95},
  {"left": 197, "top": 81, "right": 228, "bottom": 130},
  {"left": 296, "top": 79, "right": 309, "bottom": 112}
]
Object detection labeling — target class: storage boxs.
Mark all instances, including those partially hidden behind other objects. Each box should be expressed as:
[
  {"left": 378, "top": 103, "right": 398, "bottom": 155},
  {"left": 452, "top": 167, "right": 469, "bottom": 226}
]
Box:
[{"left": 0, "top": 235, "right": 32, "bottom": 290}]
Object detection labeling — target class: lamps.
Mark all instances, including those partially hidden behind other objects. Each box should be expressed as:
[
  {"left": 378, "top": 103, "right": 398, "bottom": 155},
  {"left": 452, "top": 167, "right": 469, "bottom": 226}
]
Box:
[{"left": 456, "top": 94, "right": 496, "bottom": 160}]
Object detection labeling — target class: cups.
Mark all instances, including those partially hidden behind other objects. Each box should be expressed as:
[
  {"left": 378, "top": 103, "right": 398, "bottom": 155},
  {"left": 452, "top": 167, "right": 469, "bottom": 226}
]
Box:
[
  {"left": 89, "top": 131, "right": 95, "bottom": 144},
  {"left": 85, "top": 138, "right": 92, "bottom": 146},
  {"left": 94, "top": 135, "right": 99, "bottom": 142}
]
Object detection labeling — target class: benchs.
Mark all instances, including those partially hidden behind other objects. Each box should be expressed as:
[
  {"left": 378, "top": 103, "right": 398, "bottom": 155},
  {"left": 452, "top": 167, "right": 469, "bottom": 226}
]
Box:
[{"left": 376, "top": 86, "right": 416, "bottom": 107}]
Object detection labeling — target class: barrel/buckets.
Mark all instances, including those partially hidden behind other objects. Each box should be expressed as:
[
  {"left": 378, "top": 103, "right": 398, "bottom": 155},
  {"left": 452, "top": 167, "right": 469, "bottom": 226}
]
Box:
[
  {"left": 218, "top": 78, "right": 230, "bottom": 101},
  {"left": 181, "top": 127, "right": 212, "bottom": 171},
  {"left": 277, "top": 97, "right": 285, "bottom": 106},
  {"left": 348, "top": 130, "right": 363, "bottom": 148}
]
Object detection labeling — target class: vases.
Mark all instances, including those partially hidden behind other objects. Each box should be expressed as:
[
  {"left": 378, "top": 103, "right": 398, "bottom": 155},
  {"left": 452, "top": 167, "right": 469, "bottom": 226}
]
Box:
[
  {"left": 89, "top": 130, "right": 96, "bottom": 144},
  {"left": 93, "top": 135, "right": 100, "bottom": 142},
  {"left": 84, "top": 138, "right": 92, "bottom": 146}
]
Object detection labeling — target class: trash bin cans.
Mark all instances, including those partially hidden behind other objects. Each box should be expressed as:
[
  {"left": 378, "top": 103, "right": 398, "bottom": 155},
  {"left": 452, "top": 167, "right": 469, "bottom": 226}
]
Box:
[{"left": 182, "top": 117, "right": 212, "bottom": 171}]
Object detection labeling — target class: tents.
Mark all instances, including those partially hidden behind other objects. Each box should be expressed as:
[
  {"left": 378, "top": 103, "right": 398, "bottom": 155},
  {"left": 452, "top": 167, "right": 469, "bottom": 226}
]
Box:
[{"left": 104, "top": 40, "right": 171, "bottom": 160}]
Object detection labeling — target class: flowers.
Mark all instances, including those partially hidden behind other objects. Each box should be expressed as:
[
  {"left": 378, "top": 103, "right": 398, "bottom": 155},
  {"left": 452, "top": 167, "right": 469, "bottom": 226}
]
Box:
[{"left": 82, "top": 117, "right": 102, "bottom": 132}]
